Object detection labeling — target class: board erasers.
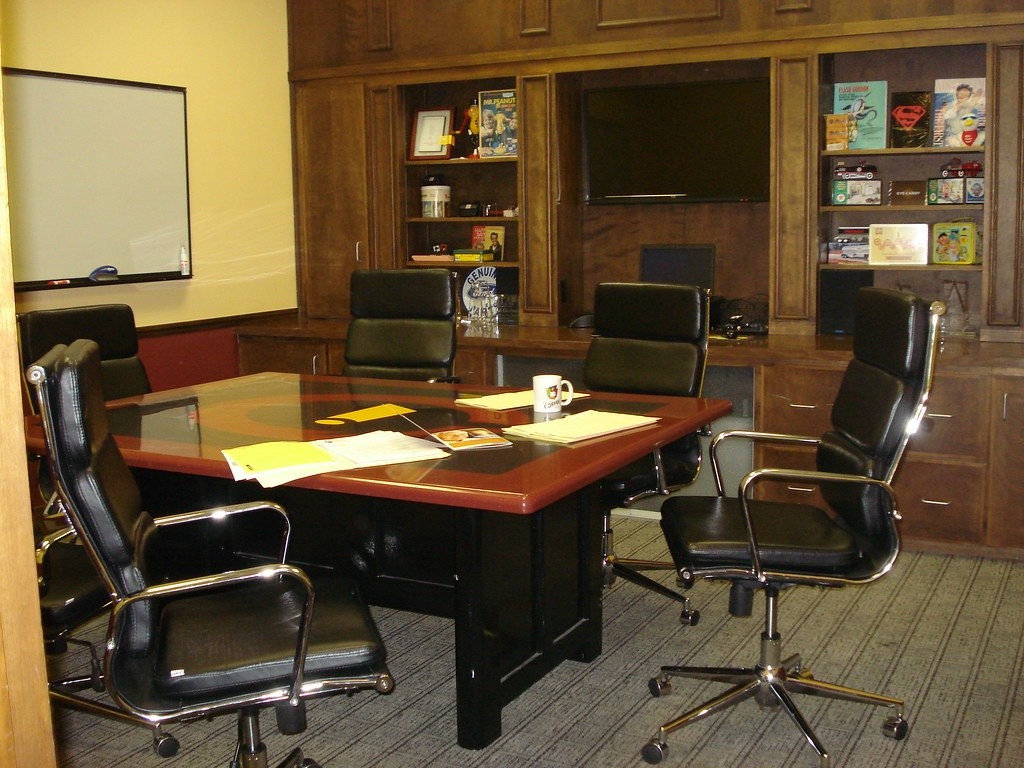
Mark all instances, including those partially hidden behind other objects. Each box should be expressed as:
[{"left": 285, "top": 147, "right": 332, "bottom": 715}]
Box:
[{"left": 89, "top": 264, "right": 117, "bottom": 281}]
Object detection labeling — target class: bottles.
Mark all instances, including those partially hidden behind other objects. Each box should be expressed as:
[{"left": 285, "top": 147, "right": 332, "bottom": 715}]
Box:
[
  {"left": 421, "top": 185, "right": 451, "bottom": 217},
  {"left": 180, "top": 245, "right": 190, "bottom": 275},
  {"left": 482, "top": 202, "right": 497, "bottom": 217},
  {"left": 480, "top": 251, "right": 484, "bottom": 262}
]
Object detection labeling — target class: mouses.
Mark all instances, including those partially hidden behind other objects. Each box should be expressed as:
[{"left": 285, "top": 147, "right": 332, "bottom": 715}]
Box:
[{"left": 724, "top": 329, "right": 737, "bottom": 339}]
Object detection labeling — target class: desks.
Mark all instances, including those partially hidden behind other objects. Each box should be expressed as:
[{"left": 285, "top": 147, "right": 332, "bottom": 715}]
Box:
[{"left": 21, "top": 370, "right": 733, "bottom": 748}]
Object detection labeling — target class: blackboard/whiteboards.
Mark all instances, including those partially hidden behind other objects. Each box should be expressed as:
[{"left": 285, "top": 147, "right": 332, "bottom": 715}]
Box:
[{"left": 0, "top": 66, "right": 193, "bottom": 292}]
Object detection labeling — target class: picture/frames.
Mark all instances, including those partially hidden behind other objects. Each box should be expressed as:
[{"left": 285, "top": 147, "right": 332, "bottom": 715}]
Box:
[{"left": 409, "top": 106, "right": 456, "bottom": 159}]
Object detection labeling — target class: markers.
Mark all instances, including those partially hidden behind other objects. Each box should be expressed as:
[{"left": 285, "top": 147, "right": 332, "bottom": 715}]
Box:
[{"left": 48, "top": 279, "right": 74, "bottom": 288}]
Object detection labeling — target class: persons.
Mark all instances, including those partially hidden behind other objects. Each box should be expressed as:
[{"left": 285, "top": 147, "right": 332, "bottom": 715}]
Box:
[
  {"left": 943, "top": 82, "right": 986, "bottom": 147},
  {"left": 436, "top": 428, "right": 494, "bottom": 444},
  {"left": 453, "top": 98, "right": 479, "bottom": 159},
  {"left": 489, "top": 232, "right": 502, "bottom": 261},
  {"left": 480, "top": 107, "right": 518, "bottom": 151}
]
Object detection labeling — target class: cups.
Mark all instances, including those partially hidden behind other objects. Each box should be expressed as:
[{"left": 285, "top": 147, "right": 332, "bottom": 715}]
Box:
[
  {"left": 532, "top": 375, "right": 573, "bottom": 413},
  {"left": 467, "top": 298, "right": 483, "bottom": 320}
]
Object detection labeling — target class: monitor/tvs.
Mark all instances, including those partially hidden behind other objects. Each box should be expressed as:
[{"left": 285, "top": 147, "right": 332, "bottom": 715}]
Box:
[
  {"left": 582, "top": 76, "right": 770, "bottom": 205},
  {"left": 638, "top": 243, "right": 715, "bottom": 295}
]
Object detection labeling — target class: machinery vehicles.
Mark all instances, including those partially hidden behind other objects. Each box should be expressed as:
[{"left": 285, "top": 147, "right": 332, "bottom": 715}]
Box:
[{"left": 831, "top": 227, "right": 870, "bottom": 243}]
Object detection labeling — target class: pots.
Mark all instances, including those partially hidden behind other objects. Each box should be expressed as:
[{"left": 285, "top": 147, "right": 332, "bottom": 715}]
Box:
[{"left": 457, "top": 200, "right": 479, "bottom": 217}]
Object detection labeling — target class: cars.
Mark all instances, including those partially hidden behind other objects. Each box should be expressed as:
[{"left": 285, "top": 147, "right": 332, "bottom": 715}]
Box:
[
  {"left": 833, "top": 160, "right": 881, "bottom": 180},
  {"left": 840, "top": 242, "right": 870, "bottom": 262},
  {"left": 939, "top": 157, "right": 983, "bottom": 179}
]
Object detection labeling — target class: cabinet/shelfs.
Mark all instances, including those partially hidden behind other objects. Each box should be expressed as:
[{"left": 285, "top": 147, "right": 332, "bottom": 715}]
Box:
[
  {"left": 235, "top": 338, "right": 498, "bottom": 385},
  {"left": 799, "top": 43, "right": 999, "bottom": 349},
  {"left": 383, "top": 62, "right": 526, "bottom": 325},
  {"left": 747, "top": 355, "right": 1024, "bottom": 566}
]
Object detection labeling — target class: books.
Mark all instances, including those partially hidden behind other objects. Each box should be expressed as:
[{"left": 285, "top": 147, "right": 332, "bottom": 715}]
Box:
[
  {"left": 430, "top": 428, "right": 513, "bottom": 451},
  {"left": 832, "top": 80, "right": 888, "bottom": 150},
  {"left": 890, "top": 92, "right": 931, "bottom": 147},
  {"left": 933, "top": 78, "right": 986, "bottom": 147}
]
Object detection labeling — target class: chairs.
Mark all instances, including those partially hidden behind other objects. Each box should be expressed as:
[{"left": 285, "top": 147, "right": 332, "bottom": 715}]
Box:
[
  {"left": 318, "top": 268, "right": 461, "bottom": 384},
  {"left": 28, "top": 342, "right": 393, "bottom": 768},
  {"left": 20, "top": 303, "right": 156, "bottom": 533},
  {"left": 641, "top": 287, "right": 945, "bottom": 768},
  {"left": 32, "top": 536, "right": 180, "bottom": 757},
  {"left": 583, "top": 282, "right": 712, "bottom": 625}
]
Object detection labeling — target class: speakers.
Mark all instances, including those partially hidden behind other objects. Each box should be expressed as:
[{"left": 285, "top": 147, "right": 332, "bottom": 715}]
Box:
[{"left": 818, "top": 268, "right": 874, "bottom": 335}]
